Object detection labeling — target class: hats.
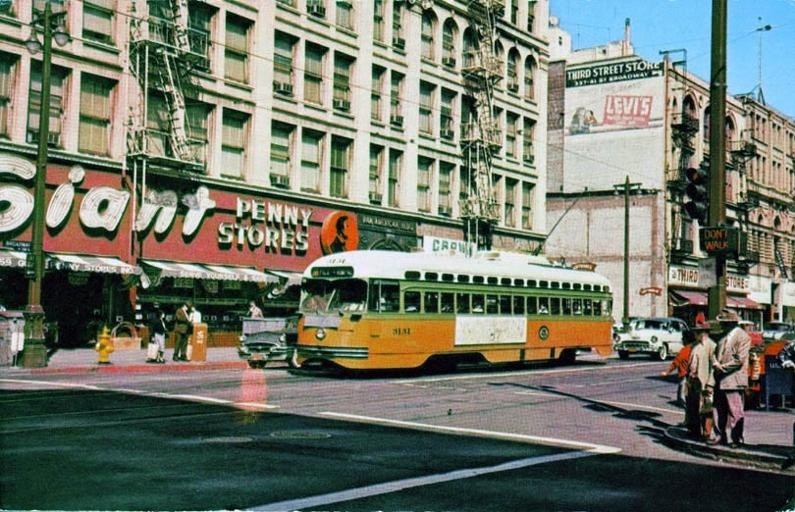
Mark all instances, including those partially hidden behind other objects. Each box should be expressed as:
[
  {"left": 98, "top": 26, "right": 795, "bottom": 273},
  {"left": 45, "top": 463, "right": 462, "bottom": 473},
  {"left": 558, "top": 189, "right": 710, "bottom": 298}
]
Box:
[
  {"left": 715, "top": 312, "right": 739, "bottom": 323},
  {"left": 691, "top": 320, "right": 715, "bottom": 332}
]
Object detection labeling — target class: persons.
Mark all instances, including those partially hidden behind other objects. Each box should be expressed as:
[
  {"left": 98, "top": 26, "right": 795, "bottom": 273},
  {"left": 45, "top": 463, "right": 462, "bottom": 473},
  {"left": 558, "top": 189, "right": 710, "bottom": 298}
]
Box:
[
  {"left": 686, "top": 323, "right": 717, "bottom": 444},
  {"left": 566, "top": 302, "right": 580, "bottom": 314},
  {"left": 712, "top": 309, "right": 751, "bottom": 449},
  {"left": 188, "top": 305, "right": 202, "bottom": 323},
  {"left": 538, "top": 305, "right": 548, "bottom": 315},
  {"left": 330, "top": 216, "right": 349, "bottom": 254},
  {"left": 376, "top": 292, "right": 388, "bottom": 311},
  {"left": 661, "top": 330, "right": 694, "bottom": 427},
  {"left": 246, "top": 299, "right": 263, "bottom": 318},
  {"left": 173, "top": 301, "right": 190, "bottom": 362},
  {"left": 152, "top": 310, "right": 167, "bottom": 364}
]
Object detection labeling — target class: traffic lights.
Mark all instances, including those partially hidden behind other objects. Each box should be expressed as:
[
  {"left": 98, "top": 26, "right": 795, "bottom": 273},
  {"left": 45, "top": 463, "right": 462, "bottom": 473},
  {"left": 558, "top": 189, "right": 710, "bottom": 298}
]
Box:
[
  {"left": 698, "top": 227, "right": 739, "bottom": 253},
  {"left": 684, "top": 164, "right": 709, "bottom": 227}
]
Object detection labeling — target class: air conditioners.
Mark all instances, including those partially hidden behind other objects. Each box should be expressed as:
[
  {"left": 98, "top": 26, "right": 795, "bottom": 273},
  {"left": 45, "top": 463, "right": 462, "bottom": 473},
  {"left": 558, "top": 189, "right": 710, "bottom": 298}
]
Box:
[
  {"left": 272, "top": 82, "right": 293, "bottom": 95},
  {"left": 333, "top": 98, "right": 351, "bottom": 112},
  {"left": 390, "top": 114, "right": 404, "bottom": 126},
  {"left": 308, "top": 4, "right": 327, "bottom": 19},
  {"left": 369, "top": 191, "right": 382, "bottom": 204},
  {"left": 269, "top": 173, "right": 290, "bottom": 187},
  {"left": 440, "top": 54, "right": 521, "bottom": 218},
  {"left": 393, "top": 36, "right": 406, "bottom": 49}
]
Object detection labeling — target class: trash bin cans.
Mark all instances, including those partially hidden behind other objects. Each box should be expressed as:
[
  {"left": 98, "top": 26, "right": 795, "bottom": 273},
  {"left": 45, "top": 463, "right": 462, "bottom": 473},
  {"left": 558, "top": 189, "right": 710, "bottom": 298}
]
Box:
[{"left": 191, "top": 323, "right": 208, "bottom": 362}]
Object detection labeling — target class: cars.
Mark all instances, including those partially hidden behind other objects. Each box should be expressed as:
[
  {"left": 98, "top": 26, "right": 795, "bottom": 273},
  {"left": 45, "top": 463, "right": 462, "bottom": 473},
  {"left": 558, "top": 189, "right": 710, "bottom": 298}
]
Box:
[
  {"left": 612, "top": 317, "right": 690, "bottom": 362},
  {"left": 759, "top": 321, "right": 795, "bottom": 342},
  {"left": 236, "top": 314, "right": 303, "bottom": 370}
]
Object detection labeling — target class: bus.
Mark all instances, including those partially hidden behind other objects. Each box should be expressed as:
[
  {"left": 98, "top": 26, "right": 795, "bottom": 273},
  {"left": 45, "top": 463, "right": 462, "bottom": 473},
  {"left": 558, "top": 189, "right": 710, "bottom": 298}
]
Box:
[{"left": 296, "top": 249, "right": 616, "bottom": 375}]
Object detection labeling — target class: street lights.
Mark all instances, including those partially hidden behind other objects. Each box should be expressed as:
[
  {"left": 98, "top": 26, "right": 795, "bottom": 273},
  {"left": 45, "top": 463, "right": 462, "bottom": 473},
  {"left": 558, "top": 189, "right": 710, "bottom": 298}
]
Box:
[
  {"left": 13, "top": 1, "right": 73, "bottom": 369},
  {"left": 613, "top": 175, "right": 646, "bottom": 328}
]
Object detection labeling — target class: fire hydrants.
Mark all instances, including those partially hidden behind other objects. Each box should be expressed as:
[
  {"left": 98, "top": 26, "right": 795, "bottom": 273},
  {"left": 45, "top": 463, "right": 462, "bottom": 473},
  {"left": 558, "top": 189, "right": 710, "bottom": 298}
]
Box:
[{"left": 94, "top": 325, "right": 115, "bottom": 364}]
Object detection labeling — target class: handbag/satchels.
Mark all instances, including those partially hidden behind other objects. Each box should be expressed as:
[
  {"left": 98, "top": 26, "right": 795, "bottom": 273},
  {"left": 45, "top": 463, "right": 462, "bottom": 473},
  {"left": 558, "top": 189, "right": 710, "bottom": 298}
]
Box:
[{"left": 147, "top": 340, "right": 160, "bottom": 360}]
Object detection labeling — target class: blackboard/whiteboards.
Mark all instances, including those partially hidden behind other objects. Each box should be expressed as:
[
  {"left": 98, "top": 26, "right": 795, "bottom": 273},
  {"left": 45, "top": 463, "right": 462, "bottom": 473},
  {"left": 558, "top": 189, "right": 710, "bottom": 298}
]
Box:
[{"left": 766, "top": 356, "right": 795, "bottom": 396}]
